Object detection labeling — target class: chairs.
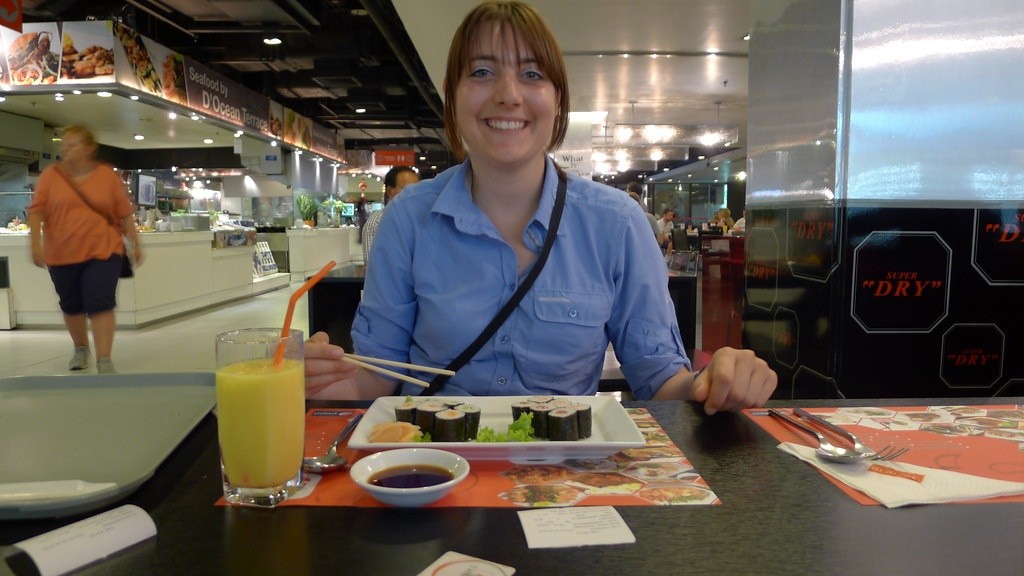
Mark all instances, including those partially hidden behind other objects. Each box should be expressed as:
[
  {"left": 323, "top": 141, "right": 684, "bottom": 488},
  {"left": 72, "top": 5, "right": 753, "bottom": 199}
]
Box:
[{"left": 668, "top": 227, "right": 701, "bottom": 275}]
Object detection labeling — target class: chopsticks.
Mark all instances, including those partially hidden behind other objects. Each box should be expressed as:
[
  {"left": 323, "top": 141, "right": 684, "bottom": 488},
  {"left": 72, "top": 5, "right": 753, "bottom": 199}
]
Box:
[{"left": 306, "top": 338, "right": 454, "bottom": 387}]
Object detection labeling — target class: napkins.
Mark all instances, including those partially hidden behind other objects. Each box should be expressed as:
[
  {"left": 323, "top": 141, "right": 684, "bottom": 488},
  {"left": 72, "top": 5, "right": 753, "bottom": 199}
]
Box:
[{"left": 775, "top": 441, "right": 1023, "bottom": 509}]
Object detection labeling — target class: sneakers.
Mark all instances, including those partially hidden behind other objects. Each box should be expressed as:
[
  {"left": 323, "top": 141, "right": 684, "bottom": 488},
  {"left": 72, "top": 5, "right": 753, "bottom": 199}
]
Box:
[
  {"left": 69, "top": 344, "right": 90, "bottom": 369},
  {"left": 96, "top": 356, "right": 116, "bottom": 373}
]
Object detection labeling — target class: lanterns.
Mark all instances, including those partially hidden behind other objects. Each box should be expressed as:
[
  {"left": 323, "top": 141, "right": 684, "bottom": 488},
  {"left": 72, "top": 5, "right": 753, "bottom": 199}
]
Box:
[{"left": 359, "top": 182, "right": 367, "bottom": 189}]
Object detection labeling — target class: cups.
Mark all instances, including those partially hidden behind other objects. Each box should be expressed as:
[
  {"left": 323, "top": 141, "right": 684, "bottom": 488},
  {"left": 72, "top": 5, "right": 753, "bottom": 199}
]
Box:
[{"left": 215, "top": 328, "right": 306, "bottom": 507}]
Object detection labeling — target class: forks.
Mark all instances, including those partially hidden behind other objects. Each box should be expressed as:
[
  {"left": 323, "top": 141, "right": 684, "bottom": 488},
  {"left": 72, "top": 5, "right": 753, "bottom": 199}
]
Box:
[{"left": 792, "top": 408, "right": 909, "bottom": 460}]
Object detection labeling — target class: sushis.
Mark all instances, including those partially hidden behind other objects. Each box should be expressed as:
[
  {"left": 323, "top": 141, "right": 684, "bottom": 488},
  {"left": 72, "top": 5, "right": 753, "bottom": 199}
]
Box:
[
  {"left": 511, "top": 395, "right": 592, "bottom": 441},
  {"left": 394, "top": 395, "right": 481, "bottom": 442}
]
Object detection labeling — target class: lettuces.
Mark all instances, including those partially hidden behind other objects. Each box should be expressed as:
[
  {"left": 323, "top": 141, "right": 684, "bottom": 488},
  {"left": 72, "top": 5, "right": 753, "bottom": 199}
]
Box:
[{"left": 413, "top": 411, "right": 539, "bottom": 443}]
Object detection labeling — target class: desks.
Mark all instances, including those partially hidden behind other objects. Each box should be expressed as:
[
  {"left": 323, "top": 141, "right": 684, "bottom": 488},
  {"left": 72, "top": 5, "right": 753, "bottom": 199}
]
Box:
[
  {"left": 256, "top": 233, "right": 289, "bottom": 274},
  {"left": 306, "top": 263, "right": 698, "bottom": 391}
]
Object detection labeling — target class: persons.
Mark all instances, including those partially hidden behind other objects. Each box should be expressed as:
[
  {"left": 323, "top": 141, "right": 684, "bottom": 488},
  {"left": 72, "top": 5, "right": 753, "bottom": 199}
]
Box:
[
  {"left": 626, "top": 182, "right": 675, "bottom": 256},
  {"left": 28, "top": 124, "right": 142, "bottom": 374},
  {"left": 711, "top": 208, "right": 745, "bottom": 233},
  {"left": 302, "top": 0, "right": 779, "bottom": 414},
  {"left": 362, "top": 165, "right": 419, "bottom": 267},
  {"left": 342, "top": 217, "right": 354, "bottom": 228}
]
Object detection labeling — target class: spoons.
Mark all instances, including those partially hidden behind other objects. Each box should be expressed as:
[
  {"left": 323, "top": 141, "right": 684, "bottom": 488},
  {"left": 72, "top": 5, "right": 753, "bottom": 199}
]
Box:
[
  {"left": 302, "top": 414, "right": 363, "bottom": 472},
  {"left": 768, "top": 408, "right": 871, "bottom": 464}
]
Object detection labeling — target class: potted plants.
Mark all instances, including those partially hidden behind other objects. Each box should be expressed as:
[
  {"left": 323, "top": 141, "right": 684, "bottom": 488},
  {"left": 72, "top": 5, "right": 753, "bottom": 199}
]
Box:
[{"left": 297, "top": 192, "right": 318, "bottom": 227}]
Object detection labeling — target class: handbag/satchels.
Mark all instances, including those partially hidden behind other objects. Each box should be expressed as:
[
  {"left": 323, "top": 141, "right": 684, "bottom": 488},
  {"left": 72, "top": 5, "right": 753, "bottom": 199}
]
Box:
[{"left": 119, "top": 253, "right": 133, "bottom": 278}]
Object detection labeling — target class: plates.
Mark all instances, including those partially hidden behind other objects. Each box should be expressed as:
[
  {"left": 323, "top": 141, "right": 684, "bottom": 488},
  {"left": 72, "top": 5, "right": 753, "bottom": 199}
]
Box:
[
  {"left": 498, "top": 407, "right": 718, "bottom": 506},
  {"left": 816, "top": 407, "right": 1023, "bottom": 452},
  {"left": 348, "top": 395, "right": 646, "bottom": 459},
  {"left": 350, "top": 448, "right": 470, "bottom": 506}
]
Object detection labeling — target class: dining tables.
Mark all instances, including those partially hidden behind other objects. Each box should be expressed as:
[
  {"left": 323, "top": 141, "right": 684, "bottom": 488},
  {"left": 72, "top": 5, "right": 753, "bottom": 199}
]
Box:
[{"left": 0, "top": 396, "right": 1024, "bottom": 576}]
